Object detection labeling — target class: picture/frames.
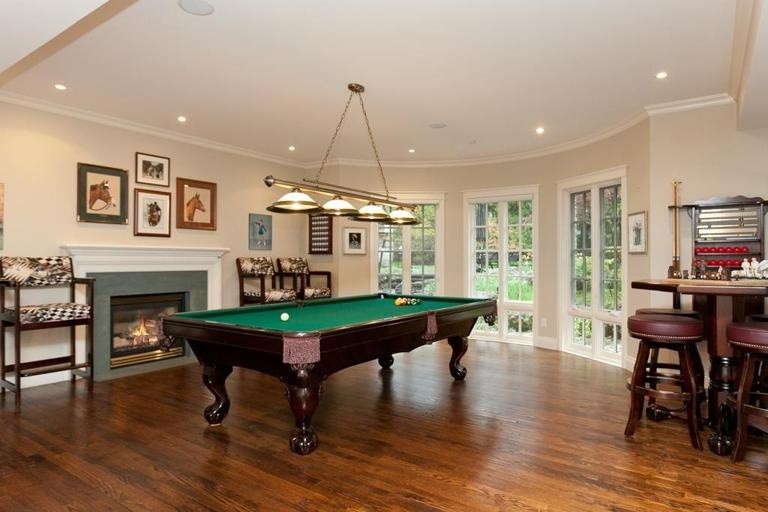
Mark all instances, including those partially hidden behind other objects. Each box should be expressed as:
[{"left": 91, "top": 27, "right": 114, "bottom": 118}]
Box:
[
  {"left": 340, "top": 227, "right": 367, "bottom": 255},
  {"left": 248, "top": 213, "right": 273, "bottom": 250},
  {"left": 626, "top": 210, "right": 647, "bottom": 256},
  {"left": 75, "top": 151, "right": 219, "bottom": 237}
]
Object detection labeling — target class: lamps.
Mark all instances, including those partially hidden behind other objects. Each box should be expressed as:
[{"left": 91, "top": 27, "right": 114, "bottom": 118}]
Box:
[{"left": 265, "top": 82, "right": 422, "bottom": 226}]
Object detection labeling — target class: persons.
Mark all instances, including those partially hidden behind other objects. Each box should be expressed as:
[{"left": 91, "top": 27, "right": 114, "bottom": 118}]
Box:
[
  {"left": 750, "top": 257, "right": 760, "bottom": 278},
  {"left": 253, "top": 218, "right": 267, "bottom": 247},
  {"left": 741, "top": 258, "right": 751, "bottom": 278}
]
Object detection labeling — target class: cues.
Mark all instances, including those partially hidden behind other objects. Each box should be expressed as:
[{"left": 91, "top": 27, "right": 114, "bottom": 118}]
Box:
[{"left": 672, "top": 183, "right": 680, "bottom": 271}]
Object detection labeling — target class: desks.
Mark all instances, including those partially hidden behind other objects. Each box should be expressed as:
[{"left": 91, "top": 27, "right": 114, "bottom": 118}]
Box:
[
  {"left": 630, "top": 274, "right": 768, "bottom": 455},
  {"left": 160, "top": 291, "right": 499, "bottom": 455}
]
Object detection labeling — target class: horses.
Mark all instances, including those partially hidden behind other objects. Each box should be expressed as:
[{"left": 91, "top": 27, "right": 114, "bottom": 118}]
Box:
[
  {"left": 186, "top": 191, "right": 206, "bottom": 222},
  {"left": 89, "top": 179, "right": 116, "bottom": 211}
]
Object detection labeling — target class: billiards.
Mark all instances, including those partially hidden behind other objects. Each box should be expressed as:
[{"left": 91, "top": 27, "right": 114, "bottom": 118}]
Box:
[
  {"left": 395, "top": 297, "right": 422, "bottom": 306},
  {"left": 279, "top": 312, "right": 290, "bottom": 322}
]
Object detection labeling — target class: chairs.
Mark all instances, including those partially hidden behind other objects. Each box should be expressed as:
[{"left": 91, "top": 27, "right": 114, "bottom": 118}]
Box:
[
  {"left": 0, "top": 257, "right": 96, "bottom": 399},
  {"left": 234, "top": 254, "right": 332, "bottom": 307}
]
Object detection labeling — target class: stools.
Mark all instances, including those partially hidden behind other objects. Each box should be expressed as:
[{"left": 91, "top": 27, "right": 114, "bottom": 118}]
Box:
[{"left": 624, "top": 302, "right": 768, "bottom": 462}]
